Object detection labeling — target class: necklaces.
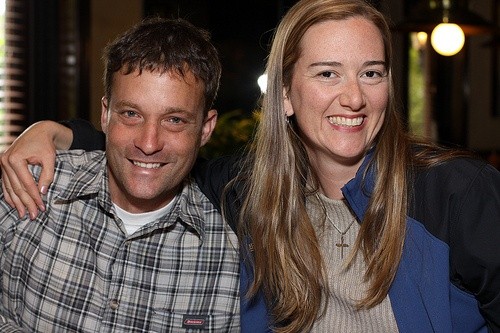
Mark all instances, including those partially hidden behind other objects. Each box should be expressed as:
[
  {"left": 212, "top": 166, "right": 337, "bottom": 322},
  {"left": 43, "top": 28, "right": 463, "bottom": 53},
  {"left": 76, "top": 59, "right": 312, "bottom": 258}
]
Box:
[{"left": 307, "top": 164, "right": 357, "bottom": 259}]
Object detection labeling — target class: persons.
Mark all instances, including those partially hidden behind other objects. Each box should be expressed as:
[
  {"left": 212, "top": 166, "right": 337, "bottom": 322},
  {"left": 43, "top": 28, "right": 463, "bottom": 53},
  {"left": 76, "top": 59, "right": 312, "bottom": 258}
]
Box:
[
  {"left": 0, "top": 0, "right": 500, "bottom": 333},
  {"left": 0, "top": 18, "right": 241, "bottom": 333}
]
